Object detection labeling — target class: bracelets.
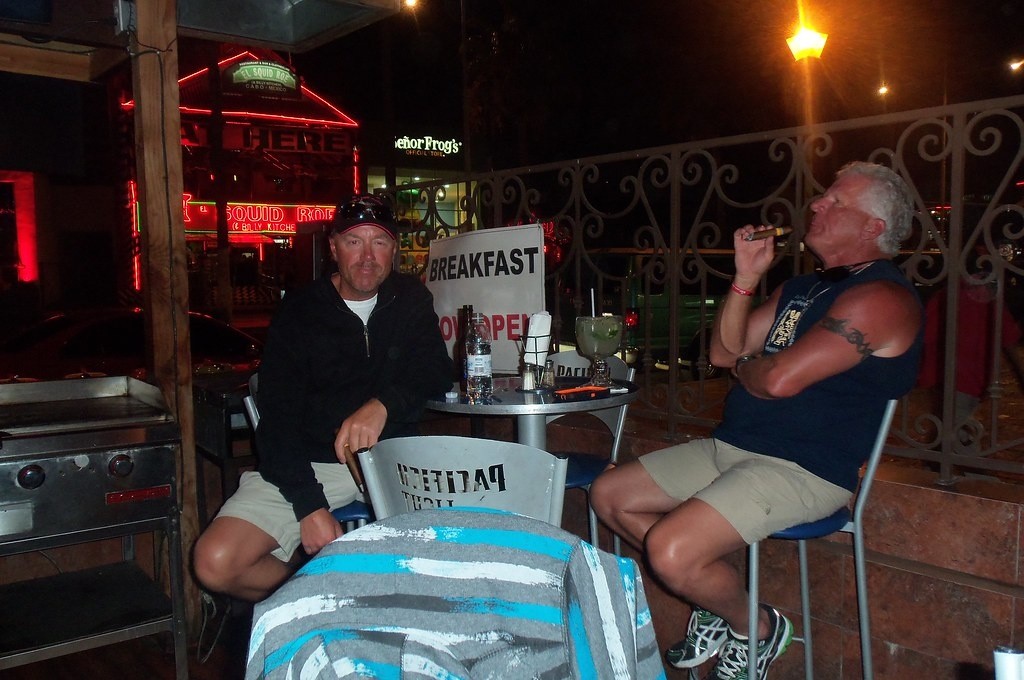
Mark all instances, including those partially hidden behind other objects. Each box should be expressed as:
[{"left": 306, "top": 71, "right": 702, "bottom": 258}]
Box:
[{"left": 730, "top": 282, "right": 755, "bottom": 296}]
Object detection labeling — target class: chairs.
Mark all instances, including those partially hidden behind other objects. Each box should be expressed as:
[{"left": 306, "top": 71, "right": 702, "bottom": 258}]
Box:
[
  {"left": 242, "top": 369, "right": 372, "bottom": 534},
  {"left": 354, "top": 435, "right": 570, "bottom": 532},
  {"left": 541, "top": 345, "right": 637, "bottom": 559},
  {"left": 686, "top": 400, "right": 899, "bottom": 680}
]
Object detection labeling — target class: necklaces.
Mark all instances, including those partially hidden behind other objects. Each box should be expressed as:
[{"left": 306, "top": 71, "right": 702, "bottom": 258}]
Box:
[{"left": 805, "top": 259, "right": 875, "bottom": 308}]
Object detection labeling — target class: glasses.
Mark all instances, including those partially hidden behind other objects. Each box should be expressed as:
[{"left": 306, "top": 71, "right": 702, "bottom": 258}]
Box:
[{"left": 336, "top": 203, "right": 393, "bottom": 222}]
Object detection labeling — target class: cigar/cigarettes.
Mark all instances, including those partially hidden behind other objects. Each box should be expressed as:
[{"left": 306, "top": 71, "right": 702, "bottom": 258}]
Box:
[
  {"left": 745, "top": 225, "right": 794, "bottom": 241},
  {"left": 344, "top": 446, "right": 364, "bottom": 492}
]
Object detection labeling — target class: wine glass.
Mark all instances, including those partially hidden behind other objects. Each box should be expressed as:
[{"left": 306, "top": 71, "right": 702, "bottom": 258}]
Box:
[{"left": 576, "top": 317, "right": 623, "bottom": 389}]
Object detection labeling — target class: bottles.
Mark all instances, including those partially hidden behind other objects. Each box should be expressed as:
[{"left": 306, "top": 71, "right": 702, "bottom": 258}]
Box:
[
  {"left": 466, "top": 312, "right": 493, "bottom": 400},
  {"left": 544, "top": 360, "right": 554, "bottom": 387},
  {"left": 459, "top": 304, "right": 474, "bottom": 392},
  {"left": 522, "top": 364, "right": 535, "bottom": 391}
]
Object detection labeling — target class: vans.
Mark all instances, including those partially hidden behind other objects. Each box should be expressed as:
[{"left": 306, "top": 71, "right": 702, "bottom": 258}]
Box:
[{"left": 581, "top": 250, "right": 824, "bottom": 381}]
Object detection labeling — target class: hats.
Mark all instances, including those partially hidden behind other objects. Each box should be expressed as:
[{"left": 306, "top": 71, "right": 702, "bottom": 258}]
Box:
[{"left": 334, "top": 193, "right": 397, "bottom": 239}]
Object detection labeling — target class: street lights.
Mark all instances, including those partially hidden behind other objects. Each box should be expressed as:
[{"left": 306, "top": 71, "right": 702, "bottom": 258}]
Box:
[{"left": 786, "top": 12, "right": 828, "bottom": 276}]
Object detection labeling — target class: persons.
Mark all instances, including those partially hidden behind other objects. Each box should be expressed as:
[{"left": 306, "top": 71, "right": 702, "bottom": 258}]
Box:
[
  {"left": 916, "top": 243, "right": 1024, "bottom": 402},
  {"left": 193, "top": 192, "right": 459, "bottom": 602},
  {"left": 588, "top": 158, "right": 926, "bottom": 680}
]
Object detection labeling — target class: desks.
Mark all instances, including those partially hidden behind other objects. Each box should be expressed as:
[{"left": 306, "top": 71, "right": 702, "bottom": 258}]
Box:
[{"left": 412, "top": 372, "right": 638, "bottom": 545}]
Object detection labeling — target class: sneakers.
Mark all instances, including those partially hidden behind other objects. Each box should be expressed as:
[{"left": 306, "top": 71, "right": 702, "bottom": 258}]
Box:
[
  {"left": 666, "top": 605, "right": 730, "bottom": 669},
  {"left": 703, "top": 603, "right": 793, "bottom": 680}
]
